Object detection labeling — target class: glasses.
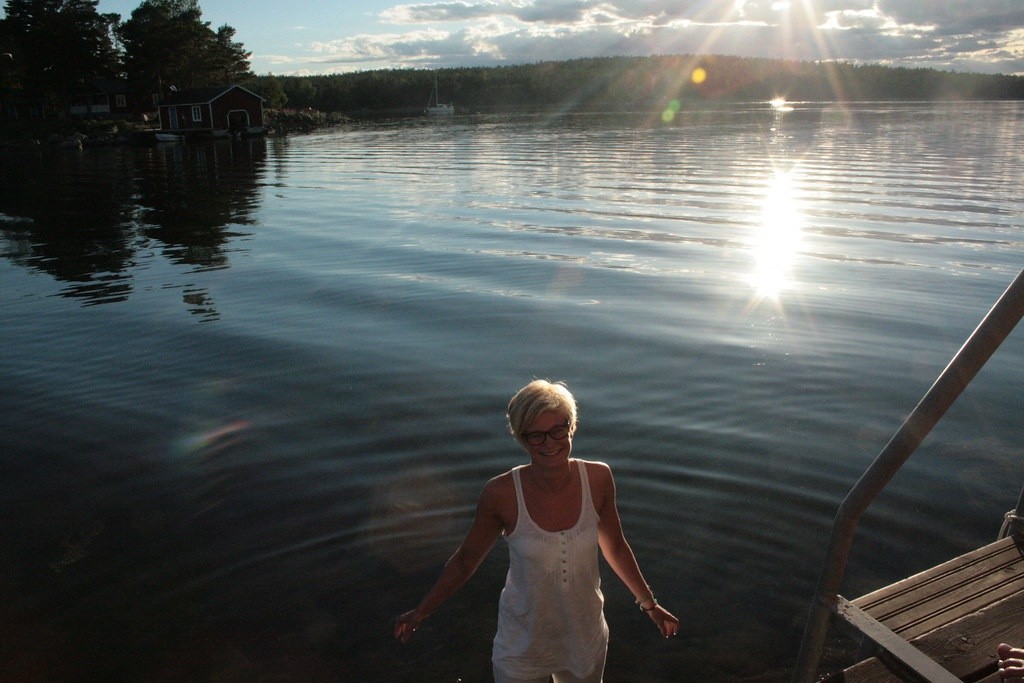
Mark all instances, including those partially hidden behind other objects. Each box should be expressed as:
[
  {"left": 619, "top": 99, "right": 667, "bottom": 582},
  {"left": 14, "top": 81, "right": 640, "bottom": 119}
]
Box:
[{"left": 521, "top": 421, "right": 570, "bottom": 445}]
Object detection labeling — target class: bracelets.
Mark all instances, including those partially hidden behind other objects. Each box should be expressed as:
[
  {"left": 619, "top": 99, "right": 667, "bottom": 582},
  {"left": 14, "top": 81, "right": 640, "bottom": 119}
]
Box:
[
  {"left": 634, "top": 590, "right": 653, "bottom": 604},
  {"left": 640, "top": 599, "right": 658, "bottom": 611}
]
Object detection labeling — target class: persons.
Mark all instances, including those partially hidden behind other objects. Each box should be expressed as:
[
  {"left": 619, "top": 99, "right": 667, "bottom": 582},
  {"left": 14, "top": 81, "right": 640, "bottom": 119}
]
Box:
[{"left": 396, "top": 379, "right": 679, "bottom": 683}]
